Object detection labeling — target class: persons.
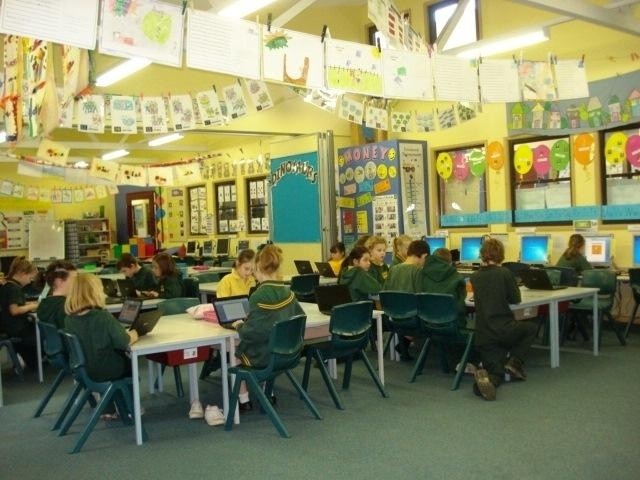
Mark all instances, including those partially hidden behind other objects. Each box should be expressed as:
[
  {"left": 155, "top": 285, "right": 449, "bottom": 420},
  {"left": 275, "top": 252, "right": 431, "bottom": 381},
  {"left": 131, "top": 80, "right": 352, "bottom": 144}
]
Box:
[
  {"left": 326, "top": 233, "right": 431, "bottom": 362},
  {"left": 61, "top": 273, "right": 150, "bottom": 423},
  {"left": 421, "top": 247, "right": 476, "bottom": 375},
  {"left": 116, "top": 249, "right": 259, "bottom": 379},
  {"left": 469, "top": 238, "right": 537, "bottom": 402},
  {"left": 554, "top": 233, "right": 594, "bottom": 330},
  {"left": 0, "top": 256, "right": 75, "bottom": 376},
  {"left": 232, "top": 245, "right": 307, "bottom": 414}
]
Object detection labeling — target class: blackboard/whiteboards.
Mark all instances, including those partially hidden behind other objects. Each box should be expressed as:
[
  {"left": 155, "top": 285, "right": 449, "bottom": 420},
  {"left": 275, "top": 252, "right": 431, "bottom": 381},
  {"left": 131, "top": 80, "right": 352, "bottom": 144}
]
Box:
[{"left": 28, "top": 219, "right": 66, "bottom": 261}]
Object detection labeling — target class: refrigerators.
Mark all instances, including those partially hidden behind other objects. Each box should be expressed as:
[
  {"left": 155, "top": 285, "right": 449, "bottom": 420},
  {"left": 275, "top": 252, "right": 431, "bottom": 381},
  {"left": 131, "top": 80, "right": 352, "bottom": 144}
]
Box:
[{"left": 28, "top": 220, "right": 65, "bottom": 271}]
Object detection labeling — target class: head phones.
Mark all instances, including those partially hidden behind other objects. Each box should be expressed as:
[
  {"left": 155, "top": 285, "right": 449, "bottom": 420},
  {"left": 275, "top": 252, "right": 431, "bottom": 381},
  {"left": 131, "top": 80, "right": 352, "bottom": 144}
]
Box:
[
  {"left": 190, "top": 240, "right": 200, "bottom": 250},
  {"left": 209, "top": 239, "right": 216, "bottom": 250}
]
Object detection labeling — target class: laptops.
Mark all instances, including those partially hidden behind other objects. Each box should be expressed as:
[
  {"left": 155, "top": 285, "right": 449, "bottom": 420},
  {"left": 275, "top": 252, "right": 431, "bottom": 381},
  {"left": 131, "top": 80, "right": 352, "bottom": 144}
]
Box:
[
  {"left": 129, "top": 309, "right": 163, "bottom": 336},
  {"left": 518, "top": 268, "right": 568, "bottom": 290},
  {"left": 211, "top": 294, "right": 249, "bottom": 329},
  {"left": 117, "top": 279, "right": 155, "bottom": 300},
  {"left": 314, "top": 262, "right": 338, "bottom": 277},
  {"left": 100, "top": 278, "right": 122, "bottom": 298},
  {"left": 31, "top": 281, "right": 51, "bottom": 312},
  {"left": 315, "top": 285, "right": 352, "bottom": 316},
  {"left": 294, "top": 261, "right": 313, "bottom": 275},
  {"left": 118, "top": 299, "right": 142, "bottom": 328}
]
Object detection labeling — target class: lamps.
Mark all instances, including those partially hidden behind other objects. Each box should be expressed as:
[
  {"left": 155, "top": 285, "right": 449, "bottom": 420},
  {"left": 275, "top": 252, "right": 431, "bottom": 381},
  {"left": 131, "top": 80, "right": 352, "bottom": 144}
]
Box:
[
  {"left": 95, "top": 57, "right": 152, "bottom": 88},
  {"left": 101, "top": 149, "right": 129, "bottom": 161},
  {"left": 456, "top": 27, "right": 550, "bottom": 63},
  {"left": 148, "top": 133, "right": 185, "bottom": 147}
]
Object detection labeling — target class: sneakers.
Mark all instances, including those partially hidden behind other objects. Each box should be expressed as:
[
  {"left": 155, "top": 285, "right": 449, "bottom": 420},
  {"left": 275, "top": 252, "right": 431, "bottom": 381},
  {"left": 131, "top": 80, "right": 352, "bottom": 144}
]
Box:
[
  {"left": 504, "top": 358, "right": 527, "bottom": 380},
  {"left": 204, "top": 404, "right": 226, "bottom": 426},
  {"left": 14, "top": 353, "right": 25, "bottom": 376},
  {"left": 239, "top": 401, "right": 252, "bottom": 415},
  {"left": 567, "top": 321, "right": 577, "bottom": 340},
  {"left": 474, "top": 370, "right": 496, "bottom": 401},
  {"left": 455, "top": 362, "right": 475, "bottom": 376},
  {"left": 189, "top": 401, "right": 204, "bottom": 420},
  {"left": 128, "top": 407, "right": 146, "bottom": 420},
  {"left": 101, "top": 412, "right": 119, "bottom": 421},
  {"left": 395, "top": 341, "right": 409, "bottom": 358},
  {"left": 267, "top": 396, "right": 276, "bottom": 406}
]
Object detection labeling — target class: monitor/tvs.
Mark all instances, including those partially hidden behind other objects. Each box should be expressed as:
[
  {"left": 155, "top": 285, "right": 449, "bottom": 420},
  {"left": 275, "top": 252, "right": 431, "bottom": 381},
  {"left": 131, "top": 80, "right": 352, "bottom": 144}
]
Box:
[
  {"left": 459, "top": 236, "right": 485, "bottom": 266},
  {"left": 186, "top": 240, "right": 197, "bottom": 256},
  {"left": 632, "top": 233, "right": 640, "bottom": 266},
  {"left": 216, "top": 238, "right": 229, "bottom": 256},
  {"left": 519, "top": 234, "right": 551, "bottom": 267},
  {"left": 581, "top": 234, "right": 613, "bottom": 266},
  {"left": 423, "top": 236, "right": 448, "bottom": 255},
  {"left": 238, "top": 240, "right": 250, "bottom": 253},
  {"left": 203, "top": 240, "right": 213, "bottom": 256}
]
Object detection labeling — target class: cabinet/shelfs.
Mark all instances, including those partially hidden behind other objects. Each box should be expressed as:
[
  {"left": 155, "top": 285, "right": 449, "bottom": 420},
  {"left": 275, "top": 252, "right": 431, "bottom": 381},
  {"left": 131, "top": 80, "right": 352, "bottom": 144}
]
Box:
[{"left": 65, "top": 217, "right": 112, "bottom": 265}]
